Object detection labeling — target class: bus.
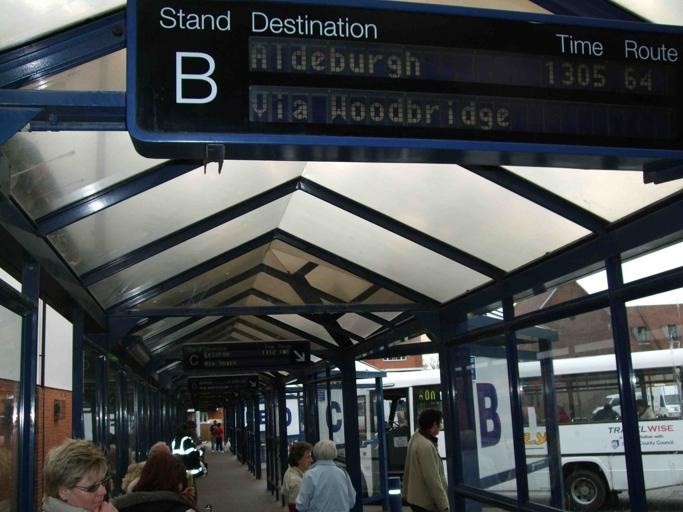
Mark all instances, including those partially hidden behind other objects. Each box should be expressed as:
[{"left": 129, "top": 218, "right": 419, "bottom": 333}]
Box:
[{"left": 359, "top": 345, "right": 682, "bottom": 512}]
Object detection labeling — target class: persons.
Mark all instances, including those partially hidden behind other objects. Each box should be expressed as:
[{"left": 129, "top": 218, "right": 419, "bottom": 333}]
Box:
[
  {"left": 400, "top": 408, "right": 450, "bottom": 511},
  {"left": 40, "top": 436, "right": 119, "bottom": 512},
  {"left": 208, "top": 420, "right": 218, "bottom": 453},
  {"left": 293, "top": 439, "right": 358, "bottom": 512},
  {"left": 213, "top": 423, "right": 225, "bottom": 454},
  {"left": 121, "top": 439, "right": 194, "bottom": 494},
  {"left": 280, "top": 441, "right": 314, "bottom": 512},
  {"left": 635, "top": 399, "right": 657, "bottom": 420},
  {"left": 590, "top": 403, "right": 620, "bottom": 420},
  {"left": 168, "top": 419, "right": 208, "bottom": 505},
  {"left": 556, "top": 401, "right": 570, "bottom": 422},
  {"left": 108, "top": 450, "right": 202, "bottom": 512}
]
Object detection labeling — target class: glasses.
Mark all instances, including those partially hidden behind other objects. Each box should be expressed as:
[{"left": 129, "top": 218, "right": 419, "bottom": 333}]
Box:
[{"left": 73, "top": 477, "right": 110, "bottom": 492}]
